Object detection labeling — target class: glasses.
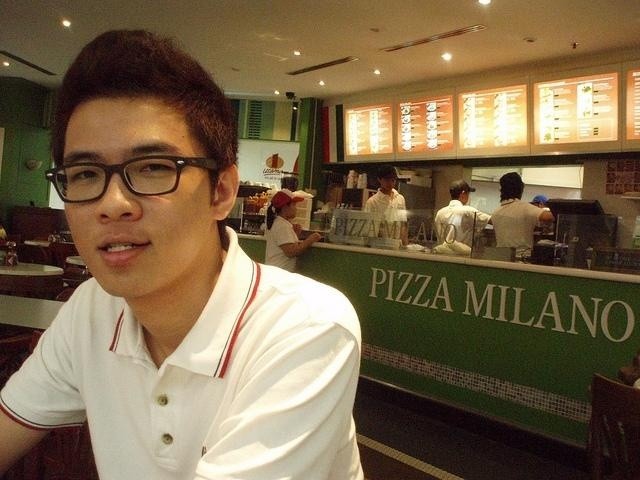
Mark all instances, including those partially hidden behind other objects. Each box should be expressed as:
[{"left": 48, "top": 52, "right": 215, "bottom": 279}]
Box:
[{"left": 42, "top": 154, "right": 220, "bottom": 203}]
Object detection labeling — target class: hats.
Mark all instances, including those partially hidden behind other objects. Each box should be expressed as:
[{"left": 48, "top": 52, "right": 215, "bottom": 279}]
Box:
[
  {"left": 271, "top": 191, "right": 304, "bottom": 209},
  {"left": 499, "top": 172, "right": 521, "bottom": 192},
  {"left": 378, "top": 164, "right": 397, "bottom": 179},
  {"left": 449, "top": 179, "right": 476, "bottom": 194},
  {"left": 528, "top": 195, "right": 548, "bottom": 204}
]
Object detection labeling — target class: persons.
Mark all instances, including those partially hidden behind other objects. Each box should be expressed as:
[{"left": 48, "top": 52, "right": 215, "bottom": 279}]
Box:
[
  {"left": 529, "top": 193, "right": 550, "bottom": 209},
  {"left": 0, "top": 25, "right": 367, "bottom": 480},
  {"left": 431, "top": 178, "right": 495, "bottom": 258},
  {"left": 489, "top": 171, "right": 556, "bottom": 269},
  {"left": 262, "top": 188, "right": 324, "bottom": 274},
  {"left": 362, "top": 164, "right": 409, "bottom": 247}
]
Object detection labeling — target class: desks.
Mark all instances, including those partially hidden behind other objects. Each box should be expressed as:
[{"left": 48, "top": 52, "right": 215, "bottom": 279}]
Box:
[{"left": 1, "top": 238, "right": 97, "bottom": 299}]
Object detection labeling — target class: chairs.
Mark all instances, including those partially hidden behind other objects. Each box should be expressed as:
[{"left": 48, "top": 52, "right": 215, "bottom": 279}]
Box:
[{"left": 578, "top": 371, "right": 640, "bottom": 480}]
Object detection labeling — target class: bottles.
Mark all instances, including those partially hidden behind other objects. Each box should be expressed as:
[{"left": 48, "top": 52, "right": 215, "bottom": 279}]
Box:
[{"left": 346, "top": 171, "right": 368, "bottom": 190}]
